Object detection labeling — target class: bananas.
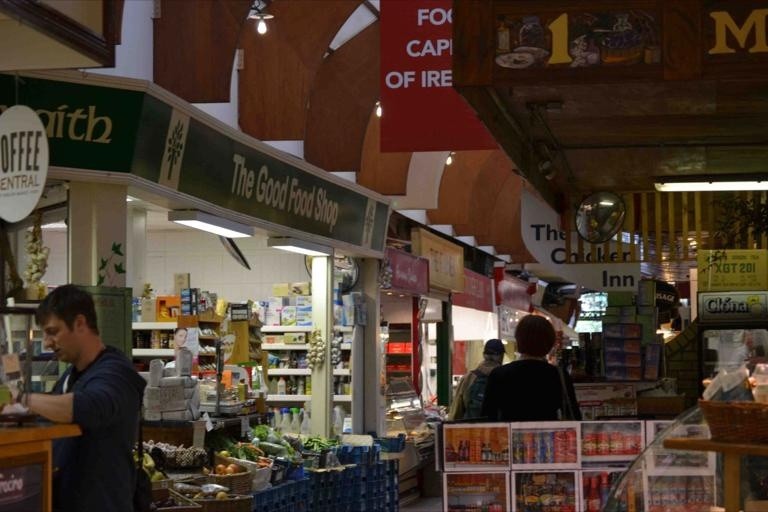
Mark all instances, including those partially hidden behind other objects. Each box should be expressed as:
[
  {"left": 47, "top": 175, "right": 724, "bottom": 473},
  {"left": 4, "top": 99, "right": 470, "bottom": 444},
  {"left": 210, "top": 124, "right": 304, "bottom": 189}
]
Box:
[{"left": 133, "top": 453, "right": 164, "bottom": 482}]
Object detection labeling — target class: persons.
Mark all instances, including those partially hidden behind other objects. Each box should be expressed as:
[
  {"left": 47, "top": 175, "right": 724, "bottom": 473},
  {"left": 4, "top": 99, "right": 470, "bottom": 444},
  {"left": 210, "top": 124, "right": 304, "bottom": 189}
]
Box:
[
  {"left": 11, "top": 283, "right": 148, "bottom": 512},
  {"left": 483, "top": 314, "right": 582, "bottom": 421},
  {"left": 448, "top": 339, "right": 505, "bottom": 420},
  {"left": 174, "top": 328, "right": 192, "bottom": 376}
]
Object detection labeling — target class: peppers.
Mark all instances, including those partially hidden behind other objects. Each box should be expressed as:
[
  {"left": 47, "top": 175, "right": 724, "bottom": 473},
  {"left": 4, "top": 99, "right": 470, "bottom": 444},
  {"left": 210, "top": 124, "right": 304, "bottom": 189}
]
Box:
[{"left": 304, "top": 437, "right": 338, "bottom": 452}]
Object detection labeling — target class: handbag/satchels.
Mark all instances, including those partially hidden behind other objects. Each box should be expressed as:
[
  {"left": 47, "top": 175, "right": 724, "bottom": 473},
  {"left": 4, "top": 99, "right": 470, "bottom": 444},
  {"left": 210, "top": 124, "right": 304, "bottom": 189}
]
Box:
[{"left": 134, "top": 468, "right": 153, "bottom": 511}]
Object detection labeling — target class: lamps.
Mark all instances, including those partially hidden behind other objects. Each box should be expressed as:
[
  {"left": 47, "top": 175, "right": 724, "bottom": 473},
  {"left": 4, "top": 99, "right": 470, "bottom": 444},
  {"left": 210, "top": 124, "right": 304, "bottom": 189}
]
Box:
[
  {"left": 654, "top": 172, "right": 767, "bottom": 193},
  {"left": 249, "top": 0, "right": 274, "bottom": 35},
  {"left": 266, "top": 236, "right": 334, "bottom": 257},
  {"left": 168, "top": 209, "right": 255, "bottom": 239}
]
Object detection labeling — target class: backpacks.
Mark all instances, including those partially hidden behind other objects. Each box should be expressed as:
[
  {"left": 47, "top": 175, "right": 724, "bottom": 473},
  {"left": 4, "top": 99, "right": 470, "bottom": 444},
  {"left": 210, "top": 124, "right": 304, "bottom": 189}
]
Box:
[{"left": 469, "top": 370, "right": 488, "bottom": 416}]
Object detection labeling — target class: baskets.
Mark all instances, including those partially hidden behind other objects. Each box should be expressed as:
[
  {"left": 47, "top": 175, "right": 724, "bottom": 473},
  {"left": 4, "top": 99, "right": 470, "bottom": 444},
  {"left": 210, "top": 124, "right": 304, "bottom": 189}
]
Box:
[
  {"left": 698, "top": 397, "right": 768, "bottom": 442},
  {"left": 208, "top": 469, "right": 252, "bottom": 494}
]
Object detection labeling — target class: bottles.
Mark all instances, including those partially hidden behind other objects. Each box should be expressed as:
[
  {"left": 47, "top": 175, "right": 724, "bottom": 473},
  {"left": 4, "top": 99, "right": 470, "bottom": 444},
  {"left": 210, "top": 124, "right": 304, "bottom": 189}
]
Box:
[
  {"left": 585, "top": 472, "right": 635, "bottom": 511},
  {"left": 237, "top": 378, "right": 248, "bottom": 402},
  {"left": 273, "top": 407, "right": 310, "bottom": 434},
  {"left": 270, "top": 376, "right": 350, "bottom": 395}
]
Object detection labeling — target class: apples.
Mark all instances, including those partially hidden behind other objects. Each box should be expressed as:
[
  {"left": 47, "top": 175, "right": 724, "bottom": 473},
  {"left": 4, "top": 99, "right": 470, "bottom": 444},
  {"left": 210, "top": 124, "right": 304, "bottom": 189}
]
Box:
[
  {"left": 216, "top": 463, "right": 239, "bottom": 474},
  {"left": 216, "top": 492, "right": 227, "bottom": 499}
]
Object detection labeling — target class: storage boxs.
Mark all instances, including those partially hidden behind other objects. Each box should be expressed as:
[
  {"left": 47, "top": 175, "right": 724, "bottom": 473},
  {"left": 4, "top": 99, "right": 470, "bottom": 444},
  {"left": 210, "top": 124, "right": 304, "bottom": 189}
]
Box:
[
  {"left": 604, "top": 277, "right": 701, "bottom": 381},
  {"left": 229, "top": 283, "right": 368, "bottom": 325}
]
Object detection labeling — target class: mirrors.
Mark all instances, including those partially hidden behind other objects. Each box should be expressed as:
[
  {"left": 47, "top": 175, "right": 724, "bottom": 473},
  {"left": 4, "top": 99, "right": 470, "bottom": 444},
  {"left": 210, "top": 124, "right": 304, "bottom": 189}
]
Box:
[
  {"left": 306, "top": 250, "right": 360, "bottom": 291},
  {"left": 577, "top": 190, "right": 627, "bottom": 243}
]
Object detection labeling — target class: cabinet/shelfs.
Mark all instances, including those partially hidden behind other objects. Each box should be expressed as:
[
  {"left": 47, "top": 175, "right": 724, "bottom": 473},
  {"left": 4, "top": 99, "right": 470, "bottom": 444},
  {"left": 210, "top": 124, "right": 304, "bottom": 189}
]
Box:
[
  {"left": 261, "top": 325, "right": 365, "bottom": 435},
  {"left": 132, "top": 320, "right": 220, "bottom": 378},
  {"left": 386, "top": 350, "right": 413, "bottom": 374},
  {"left": 439, "top": 417, "right": 718, "bottom": 512},
  {"left": 232, "top": 318, "right": 262, "bottom": 366},
  {"left": 387, "top": 389, "right": 431, "bottom": 444}
]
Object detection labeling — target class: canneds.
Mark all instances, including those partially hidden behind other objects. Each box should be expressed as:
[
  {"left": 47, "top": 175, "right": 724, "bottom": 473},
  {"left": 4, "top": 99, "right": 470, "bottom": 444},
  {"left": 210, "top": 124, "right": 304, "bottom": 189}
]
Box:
[{"left": 584, "top": 431, "right": 641, "bottom": 456}]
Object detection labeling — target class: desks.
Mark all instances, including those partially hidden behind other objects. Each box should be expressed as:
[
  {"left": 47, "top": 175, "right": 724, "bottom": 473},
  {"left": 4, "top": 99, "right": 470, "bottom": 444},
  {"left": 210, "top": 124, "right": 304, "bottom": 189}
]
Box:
[
  {"left": 0, "top": 423, "right": 84, "bottom": 512},
  {"left": 662, "top": 435, "right": 767, "bottom": 512},
  {"left": 140, "top": 411, "right": 273, "bottom": 448}
]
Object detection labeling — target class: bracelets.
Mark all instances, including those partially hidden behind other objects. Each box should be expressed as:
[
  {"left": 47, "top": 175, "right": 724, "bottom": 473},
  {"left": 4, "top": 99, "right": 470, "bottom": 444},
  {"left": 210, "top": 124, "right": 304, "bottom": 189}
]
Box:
[{"left": 15, "top": 390, "right": 29, "bottom": 407}]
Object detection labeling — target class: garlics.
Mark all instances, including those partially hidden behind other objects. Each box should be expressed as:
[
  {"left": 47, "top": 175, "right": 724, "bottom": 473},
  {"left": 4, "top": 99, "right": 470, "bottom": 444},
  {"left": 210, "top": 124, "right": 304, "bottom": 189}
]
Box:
[
  {"left": 22, "top": 230, "right": 50, "bottom": 281},
  {"left": 305, "top": 336, "right": 338, "bottom": 369}
]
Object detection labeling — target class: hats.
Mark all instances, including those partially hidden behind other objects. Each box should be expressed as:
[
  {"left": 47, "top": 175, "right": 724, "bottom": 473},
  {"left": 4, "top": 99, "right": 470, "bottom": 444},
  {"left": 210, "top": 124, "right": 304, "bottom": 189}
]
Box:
[{"left": 484, "top": 339, "right": 505, "bottom": 355}]
